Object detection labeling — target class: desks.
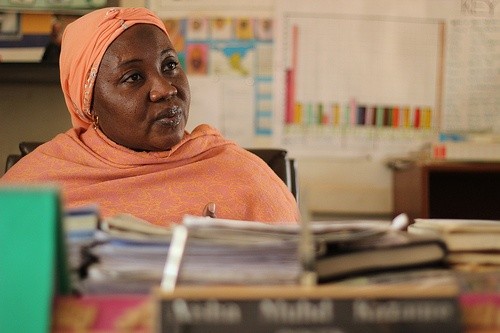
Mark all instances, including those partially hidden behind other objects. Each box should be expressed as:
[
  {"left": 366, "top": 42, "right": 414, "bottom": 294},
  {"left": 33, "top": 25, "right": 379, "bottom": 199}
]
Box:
[{"left": 386, "top": 157, "right": 500, "bottom": 224}]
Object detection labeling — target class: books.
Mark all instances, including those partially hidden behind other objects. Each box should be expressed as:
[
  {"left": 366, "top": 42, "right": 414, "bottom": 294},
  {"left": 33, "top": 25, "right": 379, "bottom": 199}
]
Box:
[{"left": 62, "top": 199, "right": 499, "bottom": 293}]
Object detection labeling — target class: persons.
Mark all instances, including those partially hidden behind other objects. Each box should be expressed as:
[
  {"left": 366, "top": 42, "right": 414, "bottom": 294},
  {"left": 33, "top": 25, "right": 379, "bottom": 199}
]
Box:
[{"left": 2, "top": 6, "right": 308, "bottom": 231}]
